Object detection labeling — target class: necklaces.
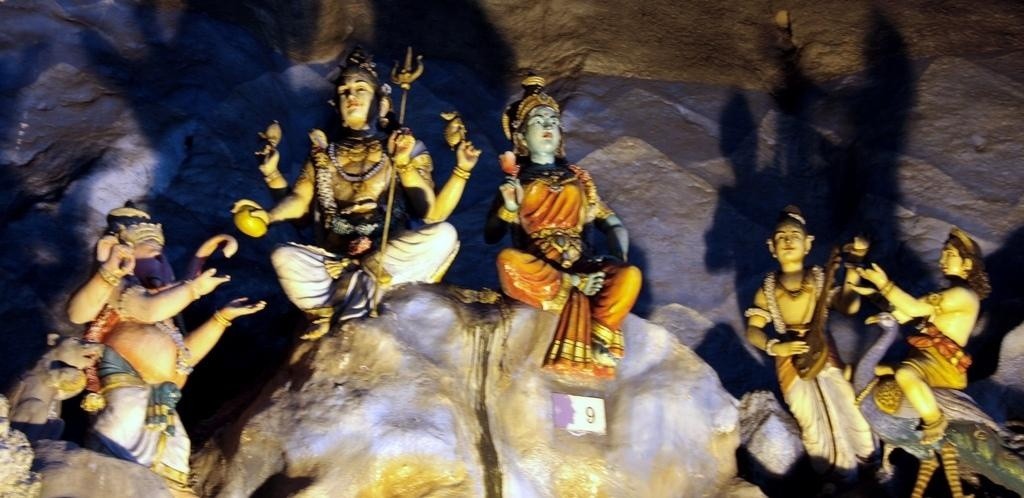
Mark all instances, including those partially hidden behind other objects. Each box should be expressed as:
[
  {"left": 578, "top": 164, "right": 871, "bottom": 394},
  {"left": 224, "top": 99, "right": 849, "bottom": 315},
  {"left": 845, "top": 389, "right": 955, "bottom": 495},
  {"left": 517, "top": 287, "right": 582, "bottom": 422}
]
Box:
[
  {"left": 774, "top": 270, "right": 811, "bottom": 298},
  {"left": 328, "top": 141, "right": 389, "bottom": 192}
]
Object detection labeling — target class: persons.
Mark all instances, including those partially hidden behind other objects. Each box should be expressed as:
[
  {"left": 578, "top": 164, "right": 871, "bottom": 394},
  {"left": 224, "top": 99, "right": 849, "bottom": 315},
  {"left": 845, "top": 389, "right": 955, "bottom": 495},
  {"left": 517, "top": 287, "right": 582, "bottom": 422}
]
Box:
[
  {"left": 487, "top": 75, "right": 643, "bottom": 375},
  {"left": 743, "top": 209, "right": 882, "bottom": 480},
  {"left": 67, "top": 196, "right": 268, "bottom": 486},
  {"left": 235, "top": 49, "right": 481, "bottom": 341},
  {"left": 845, "top": 232, "right": 992, "bottom": 444}
]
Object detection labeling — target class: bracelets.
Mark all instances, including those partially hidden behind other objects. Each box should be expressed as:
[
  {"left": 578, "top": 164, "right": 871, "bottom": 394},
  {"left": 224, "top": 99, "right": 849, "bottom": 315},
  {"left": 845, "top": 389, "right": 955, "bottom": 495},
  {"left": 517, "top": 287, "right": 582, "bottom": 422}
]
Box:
[
  {"left": 185, "top": 279, "right": 200, "bottom": 300},
  {"left": 395, "top": 162, "right": 416, "bottom": 174},
  {"left": 263, "top": 170, "right": 281, "bottom": 184},
  {"left": 452, "top": 164, "right": 471, "bottom": 180},
  {"left": 879, "top": 281, "right": 895, "bottom": 297},
  {"left": 765, "top": 336, "right": 780, "bottom": 356},
  {"left": 97, "top": 265, "right": 122, "bottom": 290},
  {"left": 495, "top": 206, "right": 518, "bottom": 224},
  {"left": 214, "top": 310, "right": 231, "bottom": 329}
]
